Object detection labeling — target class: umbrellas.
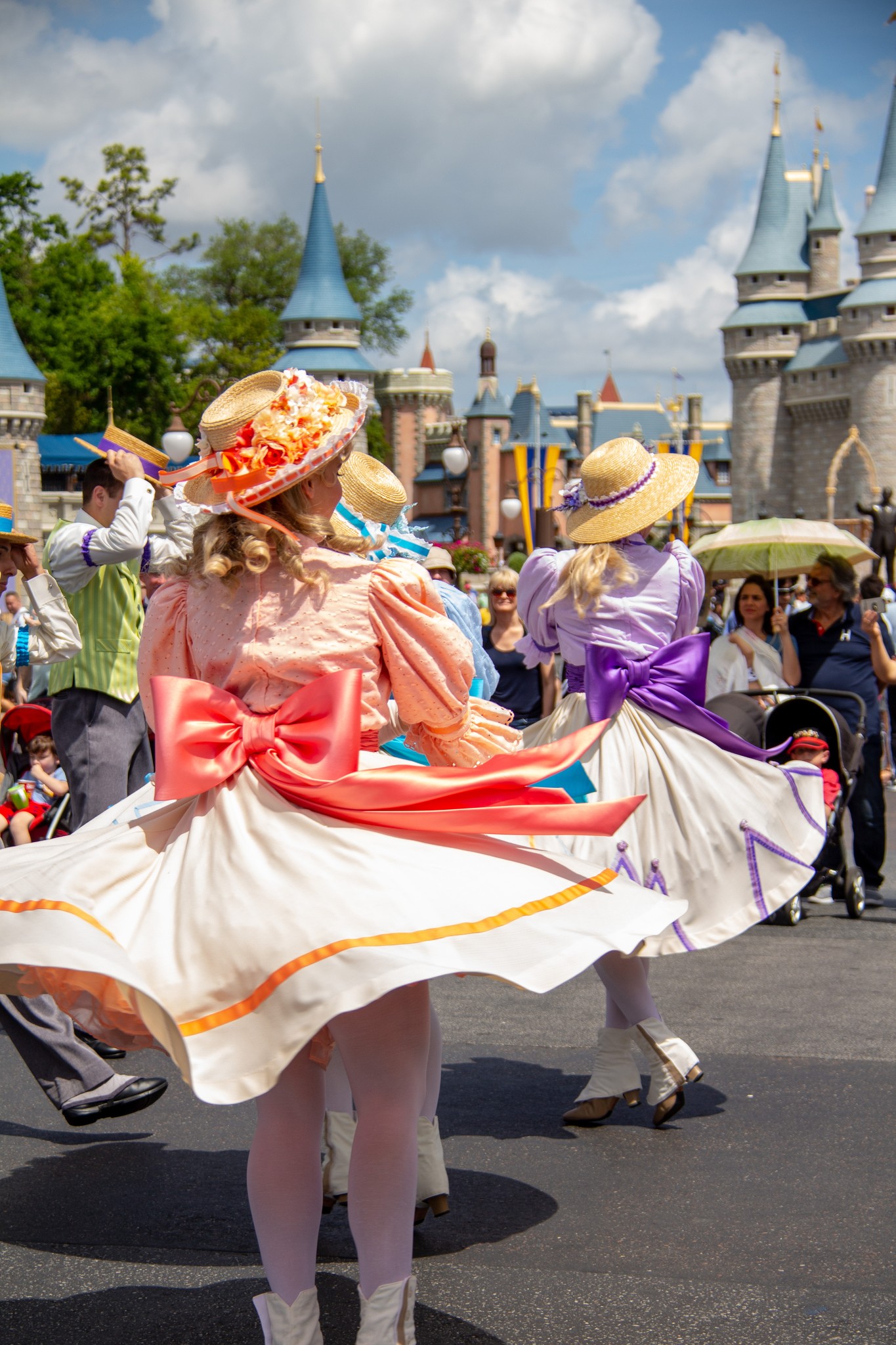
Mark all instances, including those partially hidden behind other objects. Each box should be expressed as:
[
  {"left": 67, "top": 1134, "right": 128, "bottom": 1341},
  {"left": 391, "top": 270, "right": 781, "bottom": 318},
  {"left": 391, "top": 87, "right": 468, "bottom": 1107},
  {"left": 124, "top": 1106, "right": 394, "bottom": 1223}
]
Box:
[{"left": 687, "top": 514, "right": 881, "bottom": 632}]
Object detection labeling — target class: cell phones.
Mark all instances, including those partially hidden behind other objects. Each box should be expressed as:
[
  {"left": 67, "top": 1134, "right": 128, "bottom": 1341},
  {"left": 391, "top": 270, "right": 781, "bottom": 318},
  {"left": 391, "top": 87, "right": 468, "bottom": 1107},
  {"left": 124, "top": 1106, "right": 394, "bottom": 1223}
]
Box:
[{"left": 860, "top": 597, "right": 885, "bottom": 615}]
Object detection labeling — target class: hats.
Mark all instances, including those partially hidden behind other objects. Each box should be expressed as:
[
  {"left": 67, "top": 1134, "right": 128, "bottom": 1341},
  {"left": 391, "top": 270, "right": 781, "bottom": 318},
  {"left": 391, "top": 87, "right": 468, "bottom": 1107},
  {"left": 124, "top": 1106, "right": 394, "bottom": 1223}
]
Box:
[
  {"left": 786, "top": 727, "right": 828, "bottom": 754},
  {"left": 183, "top": 367, "right": 369, "bottom": 517},
  {"left": 0, "top": 502, "right": 39, "bottom": 544},
  {"left": 322, "top": 448, "right": 433, "bottom": 561},
  {"left": 420, "top": 545, "right": 458, "bottom": 581},
  {"left": 818, "top": 552, "right": 856, "bottom": 583},
  {"left": 544, "top": 436, "right": 699, "bottom": 546},
  {"left": 73, "top": 425, "right": 176, "bottom": 490}
]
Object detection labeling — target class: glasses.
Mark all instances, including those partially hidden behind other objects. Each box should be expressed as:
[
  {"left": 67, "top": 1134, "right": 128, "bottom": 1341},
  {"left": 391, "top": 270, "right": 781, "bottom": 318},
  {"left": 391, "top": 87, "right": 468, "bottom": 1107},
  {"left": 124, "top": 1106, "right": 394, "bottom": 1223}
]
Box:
[
  {"left": 772, "top": 583, "right": 800, "bottom": 596},
  {"left": 805, "top": 572, "right": 831, "bottom": 586},
  {"left": 491, "top": 588, "right": 518, "bottom": 598}
]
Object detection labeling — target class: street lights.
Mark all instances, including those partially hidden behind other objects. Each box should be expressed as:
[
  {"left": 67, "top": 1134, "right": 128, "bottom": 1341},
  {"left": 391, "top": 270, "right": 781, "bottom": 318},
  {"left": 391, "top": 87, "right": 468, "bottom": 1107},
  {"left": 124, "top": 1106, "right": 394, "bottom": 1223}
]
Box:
[
  {"left": 440, "top": 419, "right": 471, "bottom": 542},
  {"left": 500, "top": 466, "right": 567, "bottom": 551}
]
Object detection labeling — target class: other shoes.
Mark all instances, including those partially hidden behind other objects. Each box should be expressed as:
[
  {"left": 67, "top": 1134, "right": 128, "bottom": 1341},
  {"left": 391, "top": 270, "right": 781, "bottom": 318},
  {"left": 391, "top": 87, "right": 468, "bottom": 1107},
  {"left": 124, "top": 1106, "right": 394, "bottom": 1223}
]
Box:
[
  {"left": 807, "top": 883, "right": 836, "bottom": 903},
  {"left": 864, "top": 885, "right": 884, "bottom": 907}
]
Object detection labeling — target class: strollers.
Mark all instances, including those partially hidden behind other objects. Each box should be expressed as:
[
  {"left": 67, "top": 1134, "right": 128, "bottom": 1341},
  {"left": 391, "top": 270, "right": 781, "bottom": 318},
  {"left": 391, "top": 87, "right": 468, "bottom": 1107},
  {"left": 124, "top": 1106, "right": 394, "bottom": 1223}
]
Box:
[
  {"left": 0, "top": 697, "right": 71, "bottom": 848},
  {"left": 728, "top": 688, "right": 867, "bottom": 928}
]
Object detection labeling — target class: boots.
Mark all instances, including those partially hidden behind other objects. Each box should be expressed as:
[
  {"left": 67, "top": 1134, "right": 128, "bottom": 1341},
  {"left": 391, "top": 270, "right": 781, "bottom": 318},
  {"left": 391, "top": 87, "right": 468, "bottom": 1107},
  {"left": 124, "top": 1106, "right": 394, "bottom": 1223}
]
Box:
[
  {"left": 562, "top": 1028, "right": 643, "bottom": 1123},
  {"left": 413, "top": 1115, "right": 451, "bottom": 1226},
  {"left": 354, "top": 1275, "right": 420, "bottom": 1345},
  {"left": 251, "top": 1284, "right": 324, "bottom": 1345},
  {"left": 626, "top": 1015, "right": 705, "bottom": 1127},
  {"left": 321, "top": 1110, "right": 357, "bottom": 1209}
]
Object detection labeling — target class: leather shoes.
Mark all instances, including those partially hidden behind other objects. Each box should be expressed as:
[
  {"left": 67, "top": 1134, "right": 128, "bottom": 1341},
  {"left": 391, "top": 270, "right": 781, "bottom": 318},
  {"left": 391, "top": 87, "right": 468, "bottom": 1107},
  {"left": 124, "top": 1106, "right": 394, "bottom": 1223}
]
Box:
[
  {"left": 73, "top": 1029, "right": 127, "bottom": 1059},
  {"left": 63, "top": 1076, "right": 169, "bottom": 1127}
]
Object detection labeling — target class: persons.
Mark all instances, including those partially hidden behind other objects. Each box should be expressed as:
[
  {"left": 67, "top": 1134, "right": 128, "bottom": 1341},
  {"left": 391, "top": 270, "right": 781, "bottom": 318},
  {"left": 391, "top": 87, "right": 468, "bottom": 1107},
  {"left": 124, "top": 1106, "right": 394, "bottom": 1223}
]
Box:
[
  {"left": 856, "top": 487, "right": 896, "bottom": 597},
  {"left": 515, "top": 434, "right": 711, "bottom": 1128},
  {"left": 0, "top": 441, "right": 200, "bottom": 1125},
  {"left": 321, "top": 449, "right": 503, "bottom": 1227},
  {"left": 420, "top": 543, "right": 896, "bottom": 907},
  {"left": 135, "top": 368, "right": 477, "bottom": 1345}
]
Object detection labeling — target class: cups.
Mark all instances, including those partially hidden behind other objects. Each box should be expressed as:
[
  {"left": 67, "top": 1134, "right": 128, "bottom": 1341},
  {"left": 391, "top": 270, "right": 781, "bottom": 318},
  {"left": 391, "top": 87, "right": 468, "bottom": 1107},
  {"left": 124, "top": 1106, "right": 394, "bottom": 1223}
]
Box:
[
  {"left": 18, "top": 779, "right": 36, "bottom": 799},
  {"left": 7, "top": 784, "right": 29, "bottom": 810}
]
